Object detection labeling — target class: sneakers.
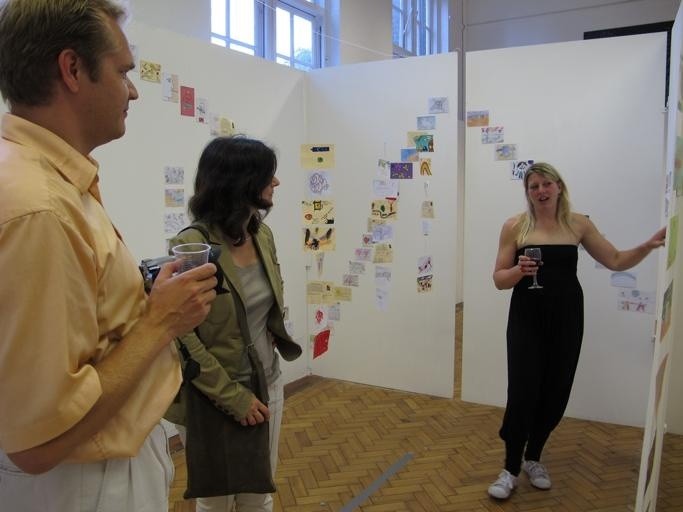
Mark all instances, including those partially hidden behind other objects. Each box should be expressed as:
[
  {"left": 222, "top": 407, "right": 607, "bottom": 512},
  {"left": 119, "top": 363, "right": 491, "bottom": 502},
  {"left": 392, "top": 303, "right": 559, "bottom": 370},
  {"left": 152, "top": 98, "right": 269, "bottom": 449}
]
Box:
[
  {"left": 522, "top": 461, "right": 552, "bottom": 489},
  {"left": 488, "top": 469, "right": 518, "bottom": 498}
]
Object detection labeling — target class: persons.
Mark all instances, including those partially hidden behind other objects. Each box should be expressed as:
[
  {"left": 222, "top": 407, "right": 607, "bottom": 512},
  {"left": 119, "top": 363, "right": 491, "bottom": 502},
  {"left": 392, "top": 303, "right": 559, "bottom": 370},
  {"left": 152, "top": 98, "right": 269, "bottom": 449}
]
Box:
[
  {"left": 487, "top": 163, "right": 666, "bottom": 499},
  {"left": 0, "top": 0, "right": 219, "bottom": 512},
  {"left": 163, "top": 134, "right": 302, "bottom": 512}
]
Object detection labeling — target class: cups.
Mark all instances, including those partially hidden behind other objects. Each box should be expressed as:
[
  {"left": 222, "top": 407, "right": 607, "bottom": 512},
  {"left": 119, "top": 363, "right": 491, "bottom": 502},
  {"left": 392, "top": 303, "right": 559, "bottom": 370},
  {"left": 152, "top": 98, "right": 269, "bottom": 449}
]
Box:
[{"left": 171, "top": 243, "right": 212, "bottom": 278}]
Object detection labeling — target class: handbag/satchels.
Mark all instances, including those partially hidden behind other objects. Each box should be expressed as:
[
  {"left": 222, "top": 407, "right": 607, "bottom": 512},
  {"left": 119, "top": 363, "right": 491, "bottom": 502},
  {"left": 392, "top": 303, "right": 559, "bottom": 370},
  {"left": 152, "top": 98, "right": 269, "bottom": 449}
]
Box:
[{"left": 183, "top": 370, "right": 276, "bottom": 500}]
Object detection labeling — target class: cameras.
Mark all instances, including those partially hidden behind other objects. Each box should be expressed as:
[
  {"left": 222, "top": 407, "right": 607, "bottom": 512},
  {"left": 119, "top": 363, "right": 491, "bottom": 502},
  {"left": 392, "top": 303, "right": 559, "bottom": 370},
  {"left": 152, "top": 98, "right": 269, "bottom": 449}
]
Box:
[{"left": 138, "top": 243, "right": 231, "bottom": 297}]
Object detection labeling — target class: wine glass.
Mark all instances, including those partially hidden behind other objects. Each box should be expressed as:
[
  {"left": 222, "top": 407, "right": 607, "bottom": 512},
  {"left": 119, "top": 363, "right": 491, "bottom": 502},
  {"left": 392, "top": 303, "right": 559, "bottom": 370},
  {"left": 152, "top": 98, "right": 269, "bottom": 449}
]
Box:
[{"left": 523, "top": 246, "right": 544, "bottom": 290}]
